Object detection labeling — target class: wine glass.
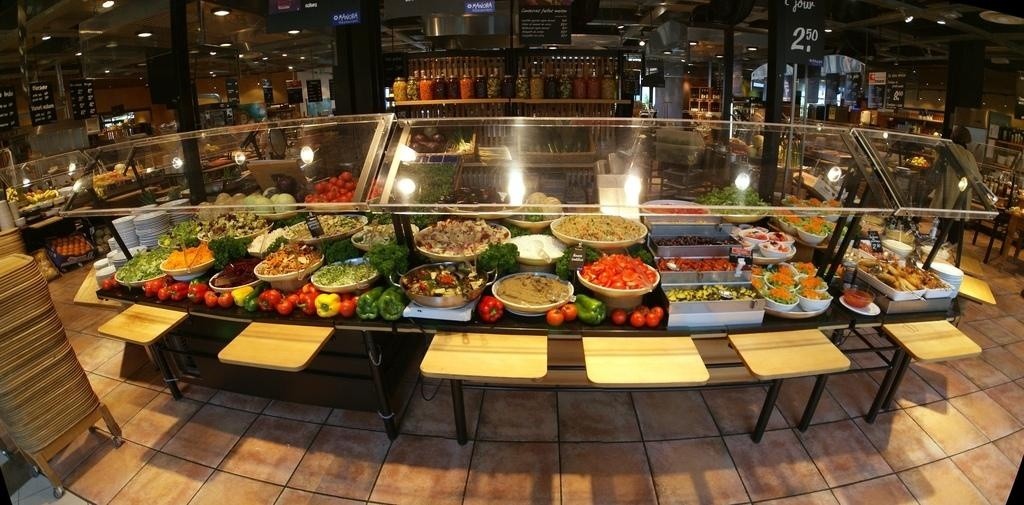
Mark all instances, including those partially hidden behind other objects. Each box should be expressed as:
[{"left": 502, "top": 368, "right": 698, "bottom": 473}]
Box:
[
  {"left": 576, "top": 262, "right": 661, "bottom": 318},
  {"left": 509, "top": 235, "right": 566, "bottom": 274},
  {"left": 252, "top": 250, "right": 325, "bottom": 293}
]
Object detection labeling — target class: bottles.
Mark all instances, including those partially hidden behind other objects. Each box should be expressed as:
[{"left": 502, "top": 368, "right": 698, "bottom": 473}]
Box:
[{"left": 699, "top": 88, "right": 720, "bottom": 111}]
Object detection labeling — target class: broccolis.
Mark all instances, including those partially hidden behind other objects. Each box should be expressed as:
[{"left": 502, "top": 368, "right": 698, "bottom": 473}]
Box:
[
  {"left": 207, "top": 236, "right": 253, "bottom": 270},
  {"left": 554, "top": 245, "right": 598, "bottom": 281},
  {"left": 324, "top": 238, "right": 359, "bottom": 265},
  {"left": 629, "top": 243, "right": 653, "bottom": 265},
  {"left": 476, "top": 243, "right": 520, "bottom": 278},
  {"left": 183, "top": 238, "right": 199, "bottom": 248},
  {"left": 363, "top": 243, "right": 410, "bottom": 286},
  {"left": 265, "top": 236, "right": 288, "bottom": 254}
]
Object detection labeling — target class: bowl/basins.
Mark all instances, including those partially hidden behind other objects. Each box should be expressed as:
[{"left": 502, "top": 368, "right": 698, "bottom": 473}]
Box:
[
  {"left": 725, "top": 221, "right": 796, "bottom": 259},
  {"left": 283, "top": 213, "right": 419, "bottom": 253},
  {"left": 842, "top": 284, "right": 876, "bottom": 307},
  {"left": 549, "top": 217, "right": 648, "bottom": 251},
  {"left": 309, "top": 258, "right": 382, "bottom": 294},
  {"left": 776, "top": 214, "right": 852, "bottom": 245},
  {"left": 414, "top": 221, "right": 511, "bottom": 262},
  {"left": 506, "top": 210, "right": 565, "bottom": 234},
  {"left": 113, "top": 218, "right": 274, "bottom": 294},
  {"left": 491, "top": 271, "right": 575, "bottom": 317},
  {"left": 741, "top": 261, "right": 832, "bottom": 311}
]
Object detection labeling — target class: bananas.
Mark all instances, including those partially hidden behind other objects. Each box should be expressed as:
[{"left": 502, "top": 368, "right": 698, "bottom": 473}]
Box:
[{"left": 7, "top": 188, "right": 60, "bottom": 204}]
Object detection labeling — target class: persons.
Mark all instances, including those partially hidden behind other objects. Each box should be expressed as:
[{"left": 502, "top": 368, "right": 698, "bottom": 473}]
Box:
[
  {"left": 933, "top": 126, "right": 972, "bottom": 245},
  {"left": 262, "top": 119, "right": 288, "bottom": 161}
]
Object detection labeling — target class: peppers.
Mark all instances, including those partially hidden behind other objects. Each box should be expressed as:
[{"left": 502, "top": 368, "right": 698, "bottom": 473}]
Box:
[
  {"left": 574, "top": 294, "right": 606, "bottom": 326},
  {"left": 477, "top": 294, "right": 503, "bottom": 322},
  {"left": 141, "top": 278, "right": 404, "bottom": 327}
]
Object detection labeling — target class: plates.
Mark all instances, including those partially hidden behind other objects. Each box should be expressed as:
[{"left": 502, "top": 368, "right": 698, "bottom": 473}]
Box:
[
  {"left": 855, "top": 265, "right": 927, "bottom": 301},
  {"left": 839, "top": 295, "right": 880, "bottom": 316},
  {"left": 921, "top": 245, "right": 949, "bottom": 260},
  {"left": 923, "top": 277, "right": 955, "bottom": 300},
  {"left": 93, "top": 245, "right": 149, "bottom": 290},
  {"left": 884, "top": 239, "right": 911, "bottom": 258},
  {"left": 640, "top": 197, "right": 711, "bottom": 215},
  {"left": 106, "top": 195, "right": 193, "bottom": 250},
  {"left": 927, "top": 262, "right": 964, "bottom": 298}
]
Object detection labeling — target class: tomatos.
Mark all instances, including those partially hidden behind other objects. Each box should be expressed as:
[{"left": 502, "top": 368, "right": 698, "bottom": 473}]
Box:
[
  {"left": 611, "top": 304, "right": 665, "bottom": 328},
  {"left": 546, "top": 303, "right": 577, "bottom": 325},
  {"left": 305, "top": 171, "right": 381, "bottom": 203},
  {"left": 101, "top": 271, "right": 118, "bottom": 292}
]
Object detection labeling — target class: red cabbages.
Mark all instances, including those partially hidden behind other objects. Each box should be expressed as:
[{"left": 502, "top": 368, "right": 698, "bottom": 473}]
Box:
[{"left": 197, "top": 175, "right": 311, "bottom": 222}]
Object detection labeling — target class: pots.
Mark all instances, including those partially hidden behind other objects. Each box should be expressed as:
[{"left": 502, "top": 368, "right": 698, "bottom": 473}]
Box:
[{"left": 387, "top": 261, "right": 499, "bottom": 310}]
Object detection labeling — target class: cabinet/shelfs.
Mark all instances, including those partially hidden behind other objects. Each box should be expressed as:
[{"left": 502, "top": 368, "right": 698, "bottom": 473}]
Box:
[{"left": 0, "top": 83, "right": 1023, "bottom": 449}]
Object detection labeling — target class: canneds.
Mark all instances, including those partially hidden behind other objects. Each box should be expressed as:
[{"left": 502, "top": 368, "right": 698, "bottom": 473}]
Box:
[{"left": 392, "top": 73, "right": 616, "bottom": 101}]
